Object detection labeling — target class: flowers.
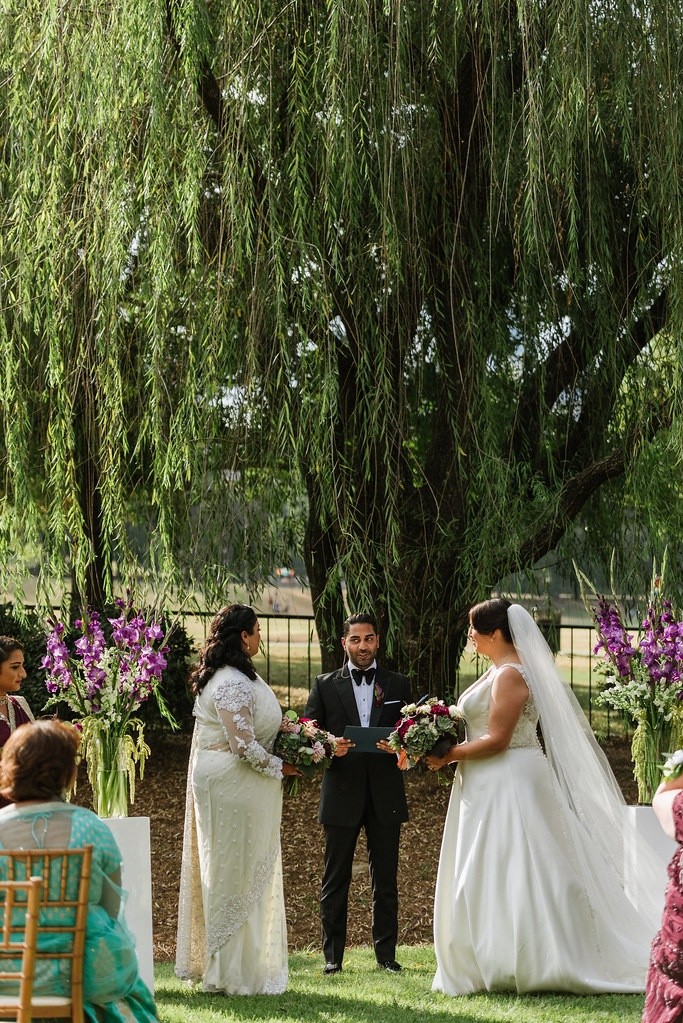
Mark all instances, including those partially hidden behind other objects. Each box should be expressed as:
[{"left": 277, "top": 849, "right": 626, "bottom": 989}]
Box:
[
  {"left": 276, "top": 710, "right": 336, "bottom": 791},
  {"left": 34, "top": 577, "right": 194, "bottom": 816},
  {"left": 572, "top": 544, "right": 683, "bottom": 806},
  {"left": 387, "top": 697, "right": 469, "bottom": 785}
]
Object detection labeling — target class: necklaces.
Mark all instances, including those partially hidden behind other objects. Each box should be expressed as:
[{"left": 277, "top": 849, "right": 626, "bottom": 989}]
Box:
[{"left": 1, "top": 695, "right": 7, "bottom": 706}]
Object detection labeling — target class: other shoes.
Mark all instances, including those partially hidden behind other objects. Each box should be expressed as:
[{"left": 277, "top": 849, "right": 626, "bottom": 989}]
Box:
[
  {"left": 323, "top": 962, "right": 343, "bottom": 975},
  {"left": 378, "top": 961, "right": 403, "bottom": 973}
]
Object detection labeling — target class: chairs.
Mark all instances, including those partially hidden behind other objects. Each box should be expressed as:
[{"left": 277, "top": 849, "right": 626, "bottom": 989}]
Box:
[{"left": 0, "top": 846, "right": 92, "bottom": 1023}]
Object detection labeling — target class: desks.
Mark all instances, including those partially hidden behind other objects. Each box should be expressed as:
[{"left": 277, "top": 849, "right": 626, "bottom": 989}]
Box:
[
  {"left": 98, "top": 817, "right": 154, "bottom": 996},
  {"left": 629, "top": 807, "right": 679, "bottom": 927}
]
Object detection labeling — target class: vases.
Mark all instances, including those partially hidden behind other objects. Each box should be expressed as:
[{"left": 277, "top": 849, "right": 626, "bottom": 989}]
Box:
[
  {"left": 95, "top": 738, "right": 128, "bottom": 818},
  {"left": 638, "top": 730, "right": 673, "bottom": 806}
]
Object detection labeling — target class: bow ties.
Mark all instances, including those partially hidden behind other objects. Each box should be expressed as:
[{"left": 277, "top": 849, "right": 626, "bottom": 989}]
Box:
[{"left": 351, "top": 667, "right": 376, "bottom": 687}]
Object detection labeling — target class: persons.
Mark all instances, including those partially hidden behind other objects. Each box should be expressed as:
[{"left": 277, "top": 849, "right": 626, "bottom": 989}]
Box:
[
  {"left": 640, "top": 772, "right": 683, "bottom": 1023},
  {"left": 302, "top": 612, "right": 414, "bottom": 975},
  {"left": 173, "top": 604, "right": 302, "bottom": 998},
  {"left": 397, "top": 599, "right": 571, "bottom": 993},
  {"left": 1, "top": 718, "right": 156, "bottom": 1023},
  {"left": 0, "top": 635, "right": 33, "bottom": 807}
]
